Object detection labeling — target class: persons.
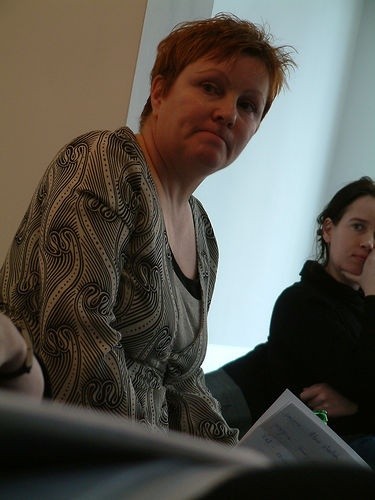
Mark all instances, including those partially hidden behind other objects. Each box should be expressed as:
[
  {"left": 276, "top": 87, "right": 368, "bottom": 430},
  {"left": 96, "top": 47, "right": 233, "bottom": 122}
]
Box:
[
  {"left": 268, "top": 175, "right": 375, "bottom": 478},
  {"left": 0, "top": 312, "right": 375, "bottom": 500},
  {"left": 0, "top": 10, "right": 296, "bottom": 448}
]
